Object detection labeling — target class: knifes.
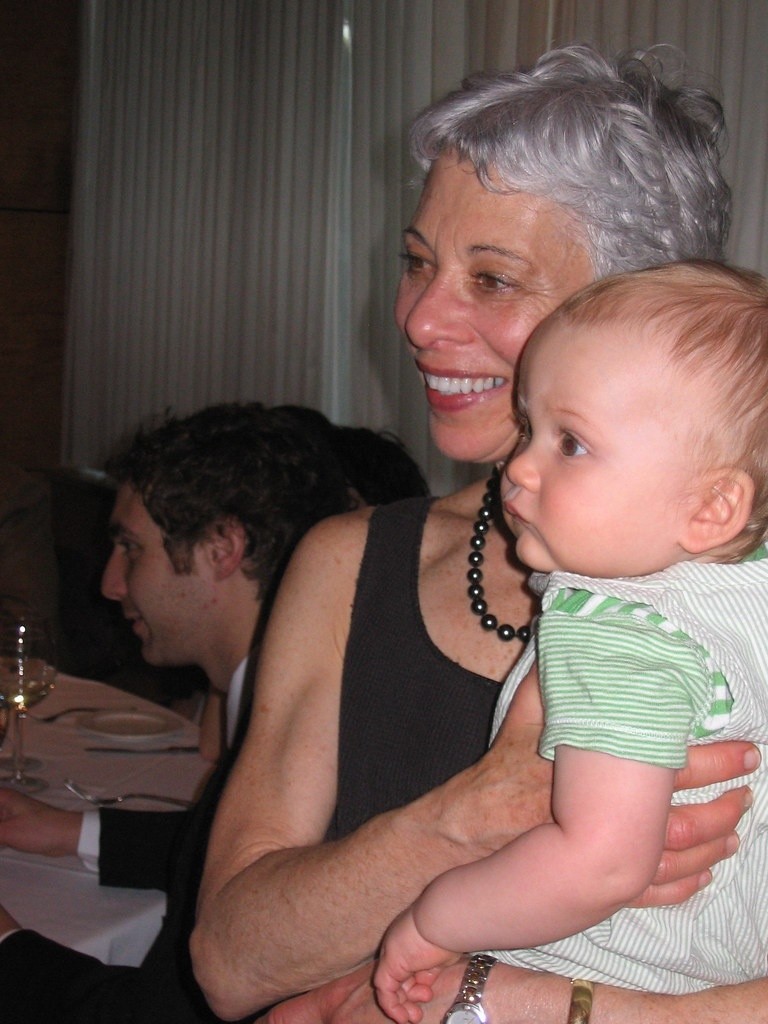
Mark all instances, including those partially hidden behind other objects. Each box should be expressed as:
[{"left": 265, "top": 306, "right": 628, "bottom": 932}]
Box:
[{"left": 85, "top": 745, "right": 201, "bottom": 755}]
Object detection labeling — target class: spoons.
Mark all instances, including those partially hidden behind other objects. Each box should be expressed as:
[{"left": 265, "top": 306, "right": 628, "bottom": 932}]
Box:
[{"left": 63, "top": 779, "right": 193, "bottom": 808}]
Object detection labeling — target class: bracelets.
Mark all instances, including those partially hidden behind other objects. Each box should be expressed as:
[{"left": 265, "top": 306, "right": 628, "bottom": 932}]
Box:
[{"left": 567, "top": 979, "right": 593, "bottom": 1024}]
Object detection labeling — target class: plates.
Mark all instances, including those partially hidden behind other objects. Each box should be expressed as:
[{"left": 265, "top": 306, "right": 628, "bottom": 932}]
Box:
[{"left": 74, "top": 709, "right": 180, "bottom": 744}]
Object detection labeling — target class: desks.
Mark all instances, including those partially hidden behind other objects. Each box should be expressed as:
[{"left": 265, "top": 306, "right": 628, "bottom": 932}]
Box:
[{"left": 0, "top": 664, "right": 217, "bottom": 967}]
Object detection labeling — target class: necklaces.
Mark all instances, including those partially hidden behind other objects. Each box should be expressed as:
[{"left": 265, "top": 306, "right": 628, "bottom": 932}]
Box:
[{"left": 467, "top": 462, "right": 532, "bottom": 642}]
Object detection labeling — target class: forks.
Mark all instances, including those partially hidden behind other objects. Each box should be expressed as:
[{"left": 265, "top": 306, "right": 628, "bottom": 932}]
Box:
[{"left": 24, "top": 707, "right": 105, "bottom": 724}]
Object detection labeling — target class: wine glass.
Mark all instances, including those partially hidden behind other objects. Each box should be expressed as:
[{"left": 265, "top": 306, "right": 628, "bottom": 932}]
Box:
[{"left": 0, "top": 609, "right": 56, "bottom": 795}]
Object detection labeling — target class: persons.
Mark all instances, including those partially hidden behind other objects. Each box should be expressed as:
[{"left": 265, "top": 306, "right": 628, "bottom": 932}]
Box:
[
  {"left": 189, "top": 44, "right": 768, "bottom": 1024},
  {"left": 0, "top": 402, "right": 432, "bottom": 1024},
  {"left": 371, "top": 257, "right": 768, "bottom": 1024}
]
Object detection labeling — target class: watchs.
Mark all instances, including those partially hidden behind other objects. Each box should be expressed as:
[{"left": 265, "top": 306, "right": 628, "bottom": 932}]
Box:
[{"left": 439, "top": 953, "right": 499, "bottom": 1024}]
